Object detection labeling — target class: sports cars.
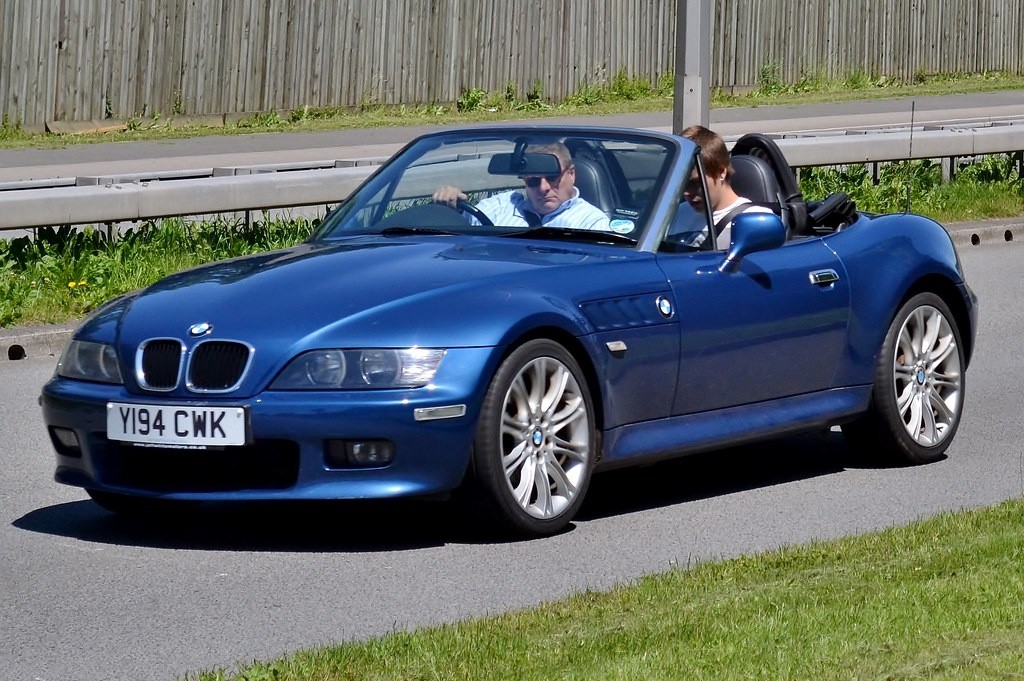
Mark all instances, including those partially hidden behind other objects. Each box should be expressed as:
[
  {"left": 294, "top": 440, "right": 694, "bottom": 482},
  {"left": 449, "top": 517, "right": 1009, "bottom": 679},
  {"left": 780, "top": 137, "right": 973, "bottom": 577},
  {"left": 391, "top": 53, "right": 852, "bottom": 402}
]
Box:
[{"left": 23, "top": 124, "right": 978, "bottom": 537}]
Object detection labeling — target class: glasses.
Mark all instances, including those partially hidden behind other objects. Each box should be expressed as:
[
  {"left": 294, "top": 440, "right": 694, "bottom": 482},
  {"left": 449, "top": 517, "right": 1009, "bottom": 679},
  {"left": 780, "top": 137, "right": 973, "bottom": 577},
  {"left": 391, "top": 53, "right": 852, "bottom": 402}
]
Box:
[{"left": 524, "top": 167, "right": 568, "bottom": 187}]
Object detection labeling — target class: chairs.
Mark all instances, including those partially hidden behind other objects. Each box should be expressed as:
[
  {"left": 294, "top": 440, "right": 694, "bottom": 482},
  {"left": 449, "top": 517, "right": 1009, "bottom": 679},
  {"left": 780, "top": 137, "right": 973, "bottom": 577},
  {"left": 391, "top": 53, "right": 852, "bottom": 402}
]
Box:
[
  {"left": 730, "top": 133, "right": 807, "bottom": 242},
  {"left": 564, "top": 138, "right": 632, "bottom": 219}
]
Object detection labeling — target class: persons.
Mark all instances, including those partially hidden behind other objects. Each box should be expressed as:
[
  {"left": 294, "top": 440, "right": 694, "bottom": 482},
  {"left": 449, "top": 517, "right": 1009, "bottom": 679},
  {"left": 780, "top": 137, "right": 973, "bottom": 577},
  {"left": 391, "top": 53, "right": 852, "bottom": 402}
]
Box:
[
  {"left": 432, "top": 143, "right": 616, "bottom": 245},
  {"left": 669, "top": 125, "right": 772, "bottom": 250}
]
